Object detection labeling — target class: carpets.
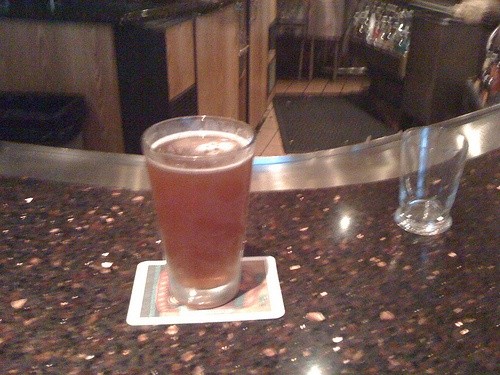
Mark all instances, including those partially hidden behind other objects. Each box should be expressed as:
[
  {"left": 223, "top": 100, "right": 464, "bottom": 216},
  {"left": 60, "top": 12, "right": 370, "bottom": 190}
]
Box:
[{"left": 272, "top": 91, "right": 396, "bottom": 154}]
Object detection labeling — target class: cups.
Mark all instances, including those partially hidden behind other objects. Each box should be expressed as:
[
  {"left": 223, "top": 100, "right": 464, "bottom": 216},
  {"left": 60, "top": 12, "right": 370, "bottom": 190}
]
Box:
[
  {"left": 398, "top": 124, "right": 470, "bottom": 236},
  {"left": 141, "top": 115, "right": 257, "bottom": 310}
]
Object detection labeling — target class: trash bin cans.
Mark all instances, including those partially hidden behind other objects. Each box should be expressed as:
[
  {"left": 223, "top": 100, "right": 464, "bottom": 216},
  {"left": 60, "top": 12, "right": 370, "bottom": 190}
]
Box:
[{"left": 0, "top": 90, "right": 84, "bottom": 151}]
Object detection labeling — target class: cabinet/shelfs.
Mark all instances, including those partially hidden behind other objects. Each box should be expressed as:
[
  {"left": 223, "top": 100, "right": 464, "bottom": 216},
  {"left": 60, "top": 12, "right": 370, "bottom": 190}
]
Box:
[{"left": 399, "top": 13, "right": 483, "bottom": 125}]
